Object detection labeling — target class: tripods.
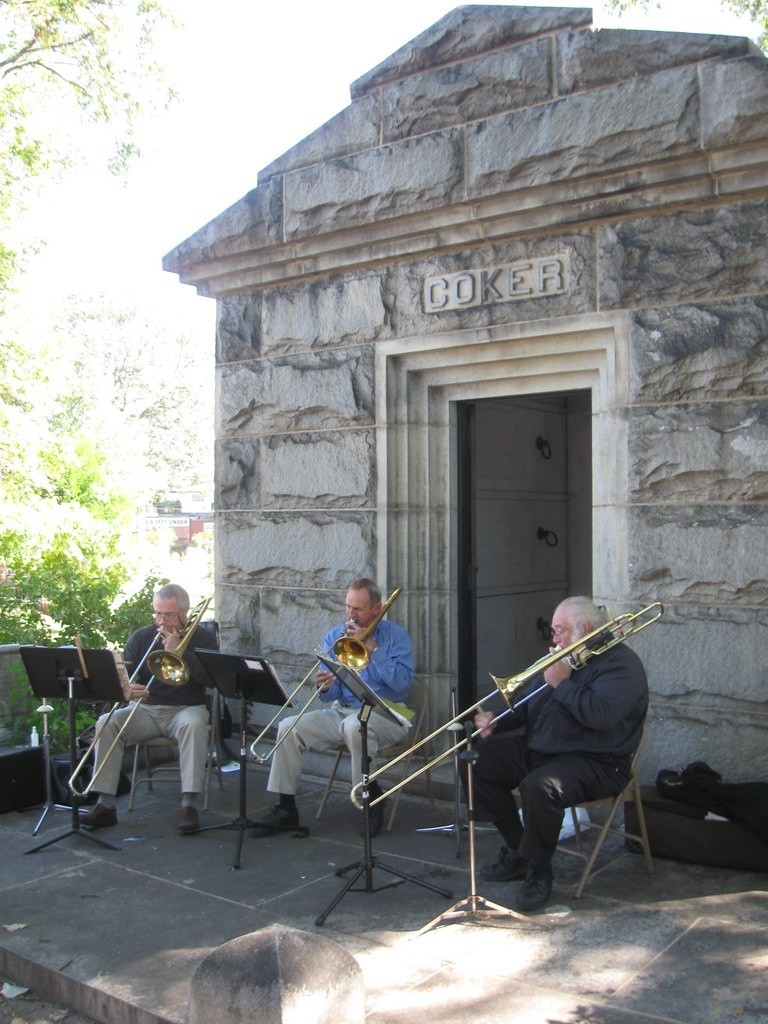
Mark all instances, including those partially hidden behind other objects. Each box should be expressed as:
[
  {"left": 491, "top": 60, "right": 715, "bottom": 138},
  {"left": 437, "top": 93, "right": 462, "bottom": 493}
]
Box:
[
  {"left": 16, "top": 674, "right": 125, "bottom": 855},
  {"left": 314, "top": 685, "right": 554, "bottom": 938},
  {"left": 180, "top": 696, "right": 309, "bottom": 872}
]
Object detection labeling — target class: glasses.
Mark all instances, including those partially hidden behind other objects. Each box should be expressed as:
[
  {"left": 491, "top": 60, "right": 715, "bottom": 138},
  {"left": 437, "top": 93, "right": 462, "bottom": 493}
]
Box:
[{"left": 152, "top": 610, "right": 183, "bottom": 622}]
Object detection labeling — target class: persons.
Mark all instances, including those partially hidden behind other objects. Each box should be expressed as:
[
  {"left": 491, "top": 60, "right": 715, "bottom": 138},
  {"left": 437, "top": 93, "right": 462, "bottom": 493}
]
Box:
[
  {"left": 458, "top": 596, "right": 650, "bottom": 911},
  {"left": 75, "top": 585, "right": 218, "bottom": 830},
  {"left": 249, "top": 578, "right": 414, "bottom": 838}
]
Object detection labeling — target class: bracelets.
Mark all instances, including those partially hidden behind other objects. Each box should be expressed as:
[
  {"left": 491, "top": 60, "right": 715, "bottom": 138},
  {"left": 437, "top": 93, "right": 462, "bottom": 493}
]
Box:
[{"left": 369, "top": 646, "right": 379, "bottom": 656}]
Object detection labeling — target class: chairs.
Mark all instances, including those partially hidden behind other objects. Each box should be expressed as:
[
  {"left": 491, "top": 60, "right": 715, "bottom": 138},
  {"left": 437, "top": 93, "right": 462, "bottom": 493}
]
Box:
[
  {"left": 555, "top": 722, "right": 653, "bottom": 900},
  {"left": 126, "top": 621, "right": 227, "bottom": 813},
  {"left": 311, "top": 674, "right": 437, "bottom": 831}
]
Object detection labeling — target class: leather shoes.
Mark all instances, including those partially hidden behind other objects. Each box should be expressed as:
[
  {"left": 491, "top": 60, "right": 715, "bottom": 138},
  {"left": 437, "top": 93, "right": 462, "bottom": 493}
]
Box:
[
  {"left": 76, "top": 804, "right": 119, "bottom": 827},
  {"left": 177, "top": 807, "right": 201, "bottom": 833},
  {"left": 480, "top": 839, "right": 528, "bottom": 881},
  {"left": 248, "top": 804, "right": 299, "bottom": 839},
  {"left": 517, "top": 860, "right": 553, "bottom": 911},
  {"left": 357, "top": 783, "right": 386, "bottom": 839}
]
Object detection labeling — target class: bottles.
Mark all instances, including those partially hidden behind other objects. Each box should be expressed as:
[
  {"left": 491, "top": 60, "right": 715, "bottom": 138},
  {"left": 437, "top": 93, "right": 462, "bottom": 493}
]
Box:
[{"left": 30, "top": 725, "right": 39, "bottom": 746}]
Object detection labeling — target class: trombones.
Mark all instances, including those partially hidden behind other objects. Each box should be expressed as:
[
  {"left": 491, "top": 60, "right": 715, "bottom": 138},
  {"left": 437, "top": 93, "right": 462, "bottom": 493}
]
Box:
[
  {"left": 349, "top": 601, "right": 665, "bottom": 813},
  {"left": 248, "top": 586, "right": 402, "bottom": 760},
  {"left": 68, "top": 598, "right": 213, "bottom": 798}
]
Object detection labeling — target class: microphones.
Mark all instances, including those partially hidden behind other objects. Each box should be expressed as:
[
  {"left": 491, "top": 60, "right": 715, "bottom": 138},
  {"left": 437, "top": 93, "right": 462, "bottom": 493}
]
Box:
[{"left": 464, "top": 719, "right": 473, "bottom": 752}]
